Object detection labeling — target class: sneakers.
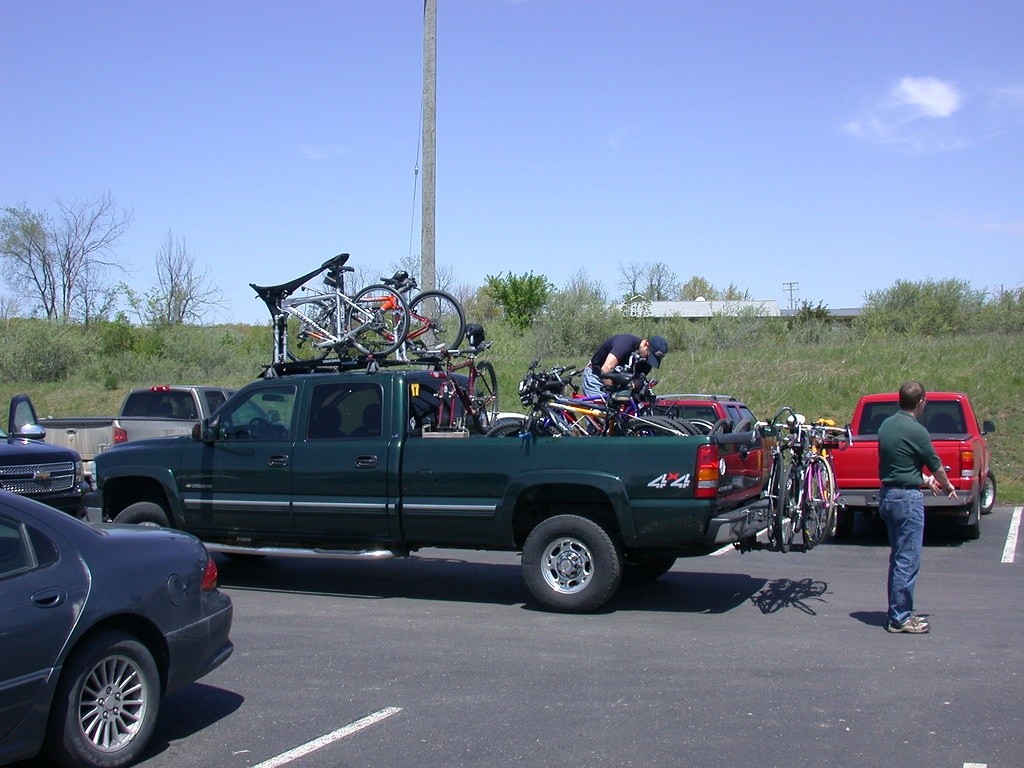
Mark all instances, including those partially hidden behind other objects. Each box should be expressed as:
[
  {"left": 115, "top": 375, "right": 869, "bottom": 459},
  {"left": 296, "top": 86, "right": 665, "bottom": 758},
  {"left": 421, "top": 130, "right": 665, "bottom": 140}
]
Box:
[{"left": 887, "top": 615, "right": 930, "bottom": 633}]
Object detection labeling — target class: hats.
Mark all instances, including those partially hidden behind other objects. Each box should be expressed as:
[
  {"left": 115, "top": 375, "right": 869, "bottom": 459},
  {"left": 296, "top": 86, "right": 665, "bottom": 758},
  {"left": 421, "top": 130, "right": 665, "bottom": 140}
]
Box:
[{"left": 647, "top": 335, "right": 668, "bottom": 369}]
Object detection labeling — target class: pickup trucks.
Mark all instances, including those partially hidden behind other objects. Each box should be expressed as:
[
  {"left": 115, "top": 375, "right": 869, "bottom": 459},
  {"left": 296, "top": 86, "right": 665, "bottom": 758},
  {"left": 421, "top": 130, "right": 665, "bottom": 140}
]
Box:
[
  {"left": 827, "top": 392, "right": 997, "bottom": 540},
  {"left": 0, "top": 394, "right": 90, "bottom": 523},
  {"left": 91, "top": 369, "right": 777, "bottom": 615},
  {"left": 37, "top": 384, "right": 283, "bottom": 492}
]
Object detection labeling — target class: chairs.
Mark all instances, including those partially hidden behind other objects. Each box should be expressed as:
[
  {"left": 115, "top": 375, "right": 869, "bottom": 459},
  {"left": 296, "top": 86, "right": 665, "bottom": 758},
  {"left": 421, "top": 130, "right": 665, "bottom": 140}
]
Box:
[
  {"left": 351, "top": 402, "right": 382, "bottom": 437},
  {"left": 312, "top": 408, "right": 346, "bottom": 438},
  {"left": 927, "top": 413, "right": 960, "bottom": 434},
  {"left": 871, "top": 415, "right": 890, "bottom": 436}
]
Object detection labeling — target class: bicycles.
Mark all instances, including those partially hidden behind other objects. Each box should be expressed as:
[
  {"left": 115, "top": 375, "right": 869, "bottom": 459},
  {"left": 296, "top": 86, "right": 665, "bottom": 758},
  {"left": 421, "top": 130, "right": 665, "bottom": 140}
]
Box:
[
  {"left": 484, "top": 355, "right": 751, "bottom": 436},
  {"left": 752, "top": 406, "right": 853, "bottom": 553},
  {"left": 413, "top": 322, "right": 498, "bottom": 436},
  {"left": 248, "top": 252, "right": 467, "bottom": 372}
]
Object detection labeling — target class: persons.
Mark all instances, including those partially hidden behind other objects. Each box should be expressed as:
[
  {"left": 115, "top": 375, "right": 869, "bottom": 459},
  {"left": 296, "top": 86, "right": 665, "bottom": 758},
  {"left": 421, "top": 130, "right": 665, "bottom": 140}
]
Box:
[
  {"left": 877, "top": 380, "right": 959, "bottom": 633},
  {"left": 581, "top": 335, "right": 668, "bottom": 425}
]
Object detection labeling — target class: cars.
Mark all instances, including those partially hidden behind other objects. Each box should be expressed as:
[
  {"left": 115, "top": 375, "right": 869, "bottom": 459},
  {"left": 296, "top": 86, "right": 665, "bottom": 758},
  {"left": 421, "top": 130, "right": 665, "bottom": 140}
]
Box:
[{"left": 0, "top": 488, "right": 234, "bottom": 768}]
